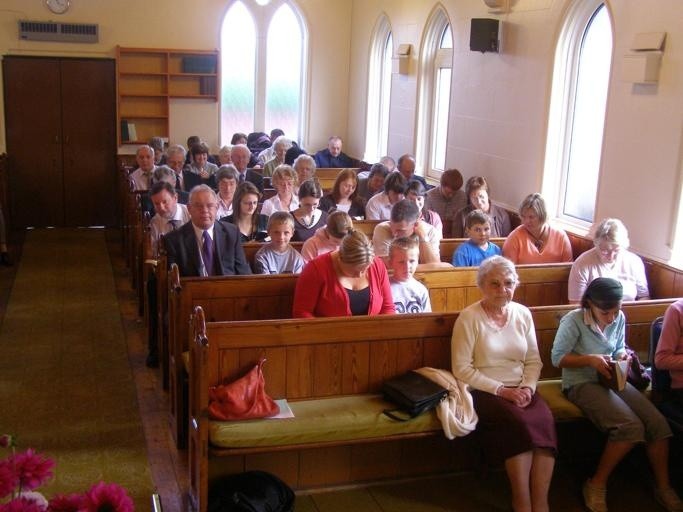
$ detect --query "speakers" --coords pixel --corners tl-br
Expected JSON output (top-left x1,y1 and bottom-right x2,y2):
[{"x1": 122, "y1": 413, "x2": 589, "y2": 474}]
[{"x1": 469, "y1": 17, "x2": 499, "y2": 52}]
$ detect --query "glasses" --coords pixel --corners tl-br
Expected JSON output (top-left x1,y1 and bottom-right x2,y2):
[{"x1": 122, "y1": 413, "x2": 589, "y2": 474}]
[
  {"x1": 487, "y1": 278, "x2": 515, "y2": 287},
  {"x1": 300, "y1": 203, "x2": 320, "y2": 210},
  {"x1": 599, "y1": 246, "x2": 618, "y2": 255}
]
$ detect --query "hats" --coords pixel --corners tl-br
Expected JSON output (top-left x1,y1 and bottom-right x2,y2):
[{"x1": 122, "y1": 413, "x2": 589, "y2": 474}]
[{"x1": 588, "y1": 278, "x2": 622, "y2": 303}]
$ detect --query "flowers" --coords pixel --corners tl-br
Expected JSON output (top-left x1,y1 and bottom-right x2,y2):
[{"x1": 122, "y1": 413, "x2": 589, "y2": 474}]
[{"x1": 0, "y1": 431, "x2": 134, "y2": 511}]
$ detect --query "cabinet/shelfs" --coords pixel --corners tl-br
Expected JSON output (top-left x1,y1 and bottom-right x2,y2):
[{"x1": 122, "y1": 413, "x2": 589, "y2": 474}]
[{"x1": 114, "y1": 43, "x2": 218, "y2": 148}]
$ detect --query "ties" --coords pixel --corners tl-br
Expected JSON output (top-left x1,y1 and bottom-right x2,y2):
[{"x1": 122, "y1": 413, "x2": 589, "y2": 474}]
[
  {"x1": 201, "y1": 230, "x2": 217, "y2": 276},
  {"x1": 239, "y1": 173, "x2": 244, "y2": 184},
  {"x1": 168, "y1": 220, "x2": 178, "y2": 232},
  {"x1": 175, "y1": 174, "x2": 181, "y2": 189}
]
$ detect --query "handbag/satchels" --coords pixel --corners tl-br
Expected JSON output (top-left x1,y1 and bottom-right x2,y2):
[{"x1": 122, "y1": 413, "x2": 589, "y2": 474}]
[
  {"x1": 207, "y1": 358, "x2": 279, "y2": 421},
  {"x1": 205, "y1": 469, "x2": 296, "y2": 512},
  {"x1": 625, "y1": 344, "x2": 652, "y2": 390},
  {"x1": 381, "y1": 370, "x2": 448, "y2": 421}
]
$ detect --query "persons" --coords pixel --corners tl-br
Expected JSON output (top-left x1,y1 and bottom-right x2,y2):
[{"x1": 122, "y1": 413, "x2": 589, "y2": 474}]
[
  {"x1": 549, "y1": 279, "x2": 683, "y2": 511},
  {"x1": 319, "y1": 154, "x2": 574, "y2": 270},
  {"x1": 451, "y1": 253, "x2": 558, "y2": 512},
  {"x1": 654, "y1": 297, "x2": 682, "y2": 425},
  {"x1": 293, "y1": 228, "x2": 396, "y2": 321},
  {"x1": 567, "y1": 216, "x2": 651, "y2": 304},
  {"x1": 382, "y1": 238, "x2": 433, "y2": 315},
  {"x1": 127, "y1": 128, "x2": 353, "y2": 277}
]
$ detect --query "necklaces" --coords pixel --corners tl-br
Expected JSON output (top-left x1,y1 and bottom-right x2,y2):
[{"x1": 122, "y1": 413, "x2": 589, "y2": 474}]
[{"x1": 483, "y1": 301, "x2": 510, "y2": 330}]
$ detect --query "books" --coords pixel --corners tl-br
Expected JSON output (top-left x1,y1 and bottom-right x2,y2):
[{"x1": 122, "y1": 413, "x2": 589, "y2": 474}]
[
  {"x1": 598, "y1": 358, "x2": 629, "y2": 391},
  {"x1": 122, "y1": 121, "x2": 137, "y2": 143},
  {"x1": 181, "y1": 55, "x2": 216, "y2": 95}
]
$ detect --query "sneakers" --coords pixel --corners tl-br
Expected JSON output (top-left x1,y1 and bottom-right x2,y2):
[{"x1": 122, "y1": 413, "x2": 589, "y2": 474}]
[
  {"x1": 653, "y1": 484, "x2": 682, "y2": 512},
  {"x1": 582, "y1": 477, "x2": 607, "y2": 512}
]
